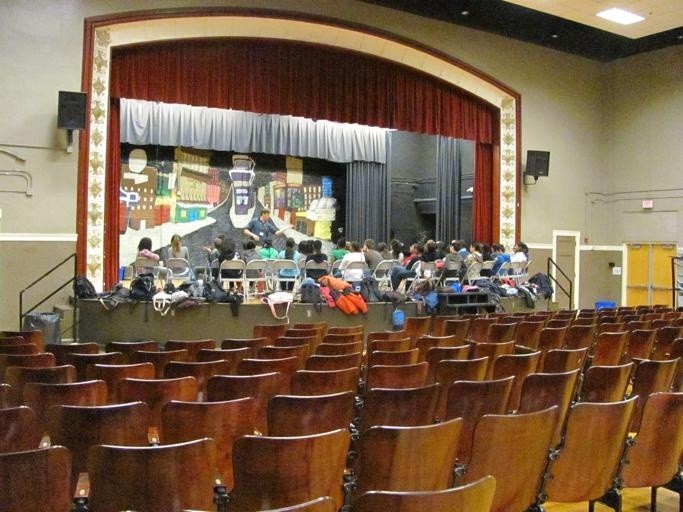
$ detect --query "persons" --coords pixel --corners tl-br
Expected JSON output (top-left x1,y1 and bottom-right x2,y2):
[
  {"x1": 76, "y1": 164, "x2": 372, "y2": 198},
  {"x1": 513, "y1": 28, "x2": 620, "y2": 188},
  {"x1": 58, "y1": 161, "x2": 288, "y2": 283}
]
[
  {"x1": 136, "y1": 235, "x2": 529, "y2": 291},
  {"x1": 243, "y1": 209, "x2": 294, "y2": 253}
]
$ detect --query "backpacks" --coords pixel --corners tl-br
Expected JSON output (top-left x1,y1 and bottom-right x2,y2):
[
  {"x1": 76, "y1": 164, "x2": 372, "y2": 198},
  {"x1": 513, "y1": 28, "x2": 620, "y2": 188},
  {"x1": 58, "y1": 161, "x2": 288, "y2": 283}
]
[{"x1": 300, "y1": 283, "x2": 323, "y2": 313}]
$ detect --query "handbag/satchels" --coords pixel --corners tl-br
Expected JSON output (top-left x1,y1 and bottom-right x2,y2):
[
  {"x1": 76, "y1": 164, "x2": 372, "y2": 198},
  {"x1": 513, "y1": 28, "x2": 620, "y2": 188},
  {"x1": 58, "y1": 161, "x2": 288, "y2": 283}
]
[
  {"x1": 261, "y1": 290, "x2": 294, "y2": 320},
  {"x1": 72, "y1": 273, "x2": 244, "y2": 317}
]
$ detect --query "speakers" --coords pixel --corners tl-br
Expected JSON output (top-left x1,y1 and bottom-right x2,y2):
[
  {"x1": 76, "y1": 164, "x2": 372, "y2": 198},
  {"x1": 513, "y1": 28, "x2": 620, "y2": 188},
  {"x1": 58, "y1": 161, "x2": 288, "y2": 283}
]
[
  {"x1": 57, "y1": 91, "x2": 87, "y2": 130},
  {"x1": 526, "y1": 150, "x2": 550, "y2": 175}
]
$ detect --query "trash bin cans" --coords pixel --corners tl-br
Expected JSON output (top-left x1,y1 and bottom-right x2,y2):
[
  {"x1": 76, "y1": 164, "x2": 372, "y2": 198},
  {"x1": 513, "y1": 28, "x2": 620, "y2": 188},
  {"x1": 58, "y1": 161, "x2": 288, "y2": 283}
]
[{"x1": 24, "y1": 312, "x2": 59, "y2": 349}]
